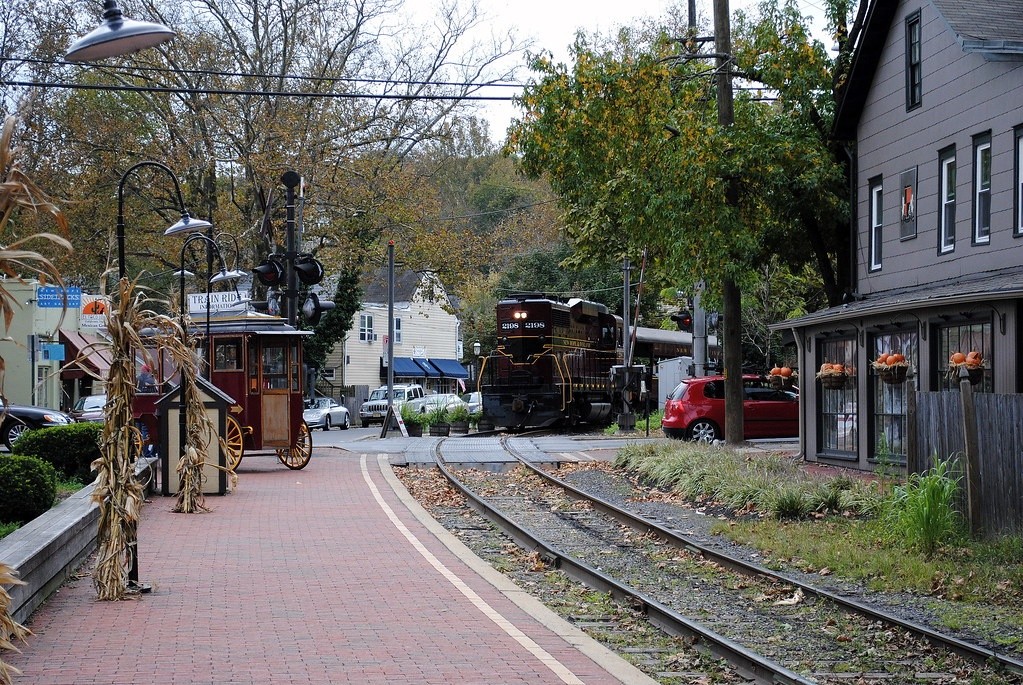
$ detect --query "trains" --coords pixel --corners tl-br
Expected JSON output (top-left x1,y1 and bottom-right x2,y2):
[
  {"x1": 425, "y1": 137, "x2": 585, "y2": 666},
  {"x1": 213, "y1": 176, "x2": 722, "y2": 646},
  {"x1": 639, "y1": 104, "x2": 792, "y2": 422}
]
[{"x1": 472, "y1": 292, "x2": 724, "y2": 434}]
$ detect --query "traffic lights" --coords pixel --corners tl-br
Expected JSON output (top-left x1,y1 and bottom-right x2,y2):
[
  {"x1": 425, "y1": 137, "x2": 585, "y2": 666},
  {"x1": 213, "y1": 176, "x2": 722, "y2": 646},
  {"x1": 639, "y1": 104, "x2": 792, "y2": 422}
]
[
  {"x1": 668, "y1": 310, "x2": 696, "y2": 332},
  {"x1": 707, "y1": 311, "x2": 724, "y2": 329},
  {"x1": 251, "y1": 258, "x2": 285, "y2": 287},
  {"x1": 297, "y1": 258, "x2": 338, "y2": 328}
]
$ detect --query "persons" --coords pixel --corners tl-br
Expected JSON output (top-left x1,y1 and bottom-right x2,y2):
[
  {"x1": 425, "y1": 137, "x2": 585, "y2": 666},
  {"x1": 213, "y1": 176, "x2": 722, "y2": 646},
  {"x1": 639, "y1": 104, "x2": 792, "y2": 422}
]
[{"x1": 138, "y1": 364, "x2": 158, "y2": 392}]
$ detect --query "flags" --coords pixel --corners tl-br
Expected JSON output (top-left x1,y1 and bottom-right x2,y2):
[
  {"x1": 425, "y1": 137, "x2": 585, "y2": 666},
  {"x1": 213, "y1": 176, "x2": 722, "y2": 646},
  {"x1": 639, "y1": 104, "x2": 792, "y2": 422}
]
[{"x1": 459, "y1": 379, "x2": 466, "y2": 392}]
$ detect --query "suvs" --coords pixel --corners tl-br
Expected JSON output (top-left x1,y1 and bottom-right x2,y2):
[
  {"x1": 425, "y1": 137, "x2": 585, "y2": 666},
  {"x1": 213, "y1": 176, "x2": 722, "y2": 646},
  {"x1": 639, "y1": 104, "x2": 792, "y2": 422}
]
[{"x1": 360, "y1": 382, "x2": 428, "y2": 424}]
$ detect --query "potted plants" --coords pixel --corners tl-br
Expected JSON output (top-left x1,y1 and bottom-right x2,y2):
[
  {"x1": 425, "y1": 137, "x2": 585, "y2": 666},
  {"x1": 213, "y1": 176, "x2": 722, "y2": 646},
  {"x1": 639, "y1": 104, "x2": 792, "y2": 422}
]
[
  {"x1": 449, "y1": 401, "x2": 473, "y2": 435},
  {"x1": 472, "y1": 408, "x2": 495, "y2": 432},
  {"x1": 400, "y1": 402, "x2": 429, "y2": 437},
  {"x1": 426, "y1": 395, "x2": 454, "y2": 436}
]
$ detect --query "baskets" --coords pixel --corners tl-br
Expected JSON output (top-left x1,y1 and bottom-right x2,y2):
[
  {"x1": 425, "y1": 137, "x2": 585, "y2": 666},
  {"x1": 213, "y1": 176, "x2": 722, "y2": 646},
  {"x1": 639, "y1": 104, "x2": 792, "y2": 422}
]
[
  {"x1": 876, "y1": 365, "x2": 908, "y2": 384},
  {"x1": 770, "y1": 376, "x2": 795, "y2": 390},
  {"x1": 822, "y1": 374, "x2": 847, "y2": 390},
  {"x1": 950, "y1": 366, "x2": 985, "y2": 385}
]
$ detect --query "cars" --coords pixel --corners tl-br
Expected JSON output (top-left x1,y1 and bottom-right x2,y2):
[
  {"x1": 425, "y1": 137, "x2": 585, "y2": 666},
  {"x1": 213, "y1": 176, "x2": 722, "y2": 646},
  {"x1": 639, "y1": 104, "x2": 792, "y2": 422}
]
[
  {"x1": 658, "y1": 374, "x2": 800, "y2": 443},
  {"x1": 0, "y1": 395, "x2": 77, "y2": 455},
  {"x1": 67, "y1": 393, "x2": 109, "y2": 425},
  {"x1": 836, "y1": 402, "x2": 858, "y2": 443},
  {"x1": 459, "y1": 391, "x2": 483, "y2": 416},
  {"x1": 111, "y1": 299, "x2": 313, "y2": 482},
  {"x1": 418, "y1": 394, "x2": 470, "y2": 418},
  {"x1": 300, "y1": 397, "x2": 351, "y2": 430}
]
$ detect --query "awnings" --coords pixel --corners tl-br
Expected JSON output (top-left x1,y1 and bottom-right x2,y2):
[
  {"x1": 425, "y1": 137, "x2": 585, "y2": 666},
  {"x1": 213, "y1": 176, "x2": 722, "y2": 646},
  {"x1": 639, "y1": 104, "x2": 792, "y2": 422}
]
[
  {"x1": 394, "y1": 357, "x2": 425, "y2": 376},
  {"x1": 428, "y1": 358, "x2": 468, "y2": 378},
  {"x1": 413, "y1": 357, "x2": 440, "y2": 377},
  {"x1": 59, "y1": 330, "x2": 116, "y2": 380}
]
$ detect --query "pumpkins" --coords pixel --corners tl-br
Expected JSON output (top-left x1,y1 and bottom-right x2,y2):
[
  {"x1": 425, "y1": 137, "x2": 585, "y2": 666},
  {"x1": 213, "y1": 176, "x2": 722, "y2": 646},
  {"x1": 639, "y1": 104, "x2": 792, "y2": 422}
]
[
  {"x1": 951, "y1": 352, "x2": 982, "y2": 365},
  {"x1": 771, "y1": 363, "x2": 792, "y2": 376},
  {"x1": 821, "y1": 363, "x2": 844, "y2": 372},
  {"x1": 877, "y1": 353, "x2": 905, "y2": 365}
]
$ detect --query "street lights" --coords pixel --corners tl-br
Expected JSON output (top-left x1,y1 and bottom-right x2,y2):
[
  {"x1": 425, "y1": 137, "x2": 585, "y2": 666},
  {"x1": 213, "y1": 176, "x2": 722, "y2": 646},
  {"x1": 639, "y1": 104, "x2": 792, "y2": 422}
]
[
  {"x1": 173, "y1": 230, "x2": 250, "y2": 495},
  {"x1": 114, "y1": 160, "x2": 215, "y2": 335},
  {"x1": 473, "y1": 338, "x2": 482, "y2": 390}
]
